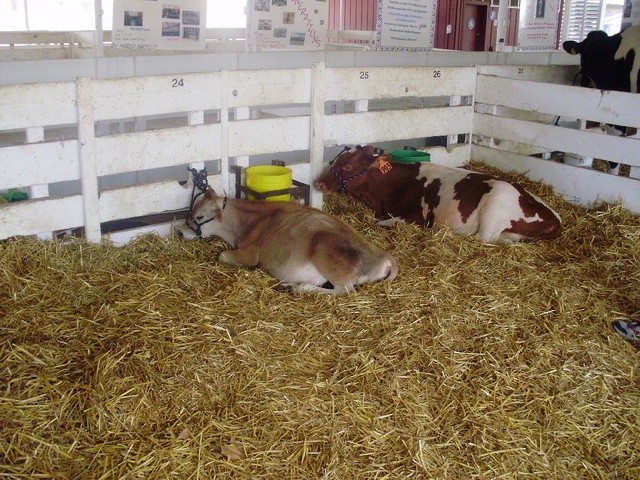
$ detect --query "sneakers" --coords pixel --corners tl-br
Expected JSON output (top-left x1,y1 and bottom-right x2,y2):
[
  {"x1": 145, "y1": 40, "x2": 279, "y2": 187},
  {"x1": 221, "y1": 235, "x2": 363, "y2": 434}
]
[{"x1": 613, "y1": 318, "x2": 640, "y2": 341}]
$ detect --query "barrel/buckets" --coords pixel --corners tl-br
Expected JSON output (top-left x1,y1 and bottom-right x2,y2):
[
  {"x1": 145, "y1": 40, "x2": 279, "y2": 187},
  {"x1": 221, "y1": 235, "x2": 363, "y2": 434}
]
[
  {"x1": 390, "y1": 151, "x2": 431, "y2": 163},
  {"x1": 246, "y1": 165, "x2": 292, "y2": 201}
]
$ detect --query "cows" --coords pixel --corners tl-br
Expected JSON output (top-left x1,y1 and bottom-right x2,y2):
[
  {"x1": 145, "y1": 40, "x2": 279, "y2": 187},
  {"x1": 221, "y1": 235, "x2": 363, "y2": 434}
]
[
  {"x1": 177, "y1": 183, "x2": 400, "y2": 297},
  {"x1": 313, "y1": 142, "x2": 563, "y2": 244},
  {"x1": 562, "y1": 24, "x2": 640, "y2": 176}
]
